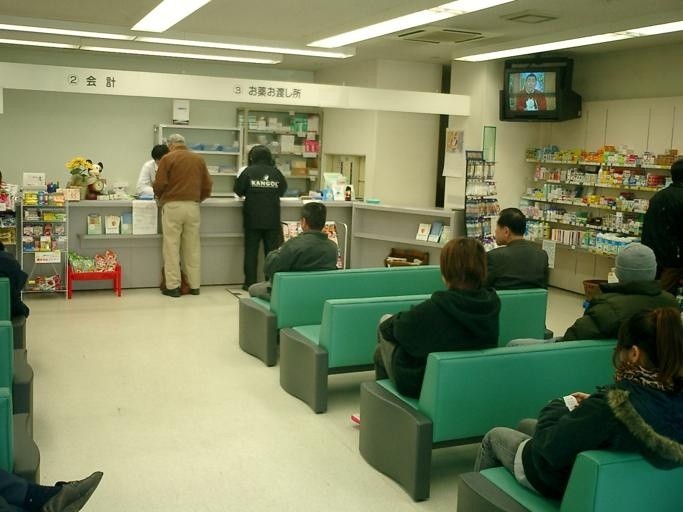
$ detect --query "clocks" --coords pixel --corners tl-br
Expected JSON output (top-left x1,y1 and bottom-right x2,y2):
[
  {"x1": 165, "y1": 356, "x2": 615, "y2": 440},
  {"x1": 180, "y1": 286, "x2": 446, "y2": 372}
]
[{"x1": 85, "y1": 159, "x2": 107, "y2": 200}]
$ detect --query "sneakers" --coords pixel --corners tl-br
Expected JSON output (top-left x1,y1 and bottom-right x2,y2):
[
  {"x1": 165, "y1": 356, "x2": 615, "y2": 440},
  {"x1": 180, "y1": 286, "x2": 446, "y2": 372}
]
[
  {"x1": 190, "y1": 288, "x2": 200, "y2": 295},
  {"x1": 161, "y1": 288, "x2": 182, "y2": 297}
]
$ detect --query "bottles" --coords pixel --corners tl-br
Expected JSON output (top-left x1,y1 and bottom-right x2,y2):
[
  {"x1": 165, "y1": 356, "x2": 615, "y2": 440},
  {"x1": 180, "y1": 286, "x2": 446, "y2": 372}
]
[
  {"x1": 544, "y1": 223, "x2": 551, "y2": 239},
  {"x1": 38, "y1": 191, "x2": 43, "y2": 204},
  {"x1": 345, "y1": 186, "x2": 352, "y2": 200},
  {"x1": 44, "y1": 193, "x2": 49, "y2": 204},
  {"x1": 539, "y1": 223, "x2": 544, "y2": 240}
]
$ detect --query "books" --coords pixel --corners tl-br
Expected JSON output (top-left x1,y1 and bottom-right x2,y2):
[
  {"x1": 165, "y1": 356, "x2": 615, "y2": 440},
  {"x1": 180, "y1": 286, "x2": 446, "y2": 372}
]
[{"x1": 416, "y1": 221, "x2": 450, "y2": 243}]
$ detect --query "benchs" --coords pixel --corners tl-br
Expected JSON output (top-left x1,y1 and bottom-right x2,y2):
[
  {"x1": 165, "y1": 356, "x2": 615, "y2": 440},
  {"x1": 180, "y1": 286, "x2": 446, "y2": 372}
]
[
  {"x1": 455, "y1": 442, "x2": 683, "y2": 512},
  {"x1": 0, "y1": 321, "x2": 33, "y2": 415},
  {"x1": 279, "y1": 287, "x2": 554, "y2": 414},
  {"x1": 0, "y1": 277, "x2": 26, "y2": 350},
  {"x1": 358, "y1": 338, "x2": 623, "y2": 503},
  {"x1": 238, "y1": 265, "x2": 451, "y2": 367},
  {"x1": 0, "y1": 386, "x2": 41, "y2": 487}
]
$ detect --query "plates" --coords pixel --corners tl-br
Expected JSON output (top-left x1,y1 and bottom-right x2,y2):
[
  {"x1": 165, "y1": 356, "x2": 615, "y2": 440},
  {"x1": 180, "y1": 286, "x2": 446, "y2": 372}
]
[{"x1": 365, "y1": 199, "x2": 380, "y2": 203}]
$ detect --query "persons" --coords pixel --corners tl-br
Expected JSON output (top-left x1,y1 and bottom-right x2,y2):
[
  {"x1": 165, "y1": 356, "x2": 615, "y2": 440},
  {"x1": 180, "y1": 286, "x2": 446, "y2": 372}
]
[
  {"x1": 641, "y1": 159, "x2": 683, "y2": 295},
  {"x1": 153, "y1": 134, "x2": 213, "y2": 297},
  {"x1": 351, "y1": 235, "x2": 502, "y2": 424},
  {"x1": 473, "y1": 307, "x2": 683, "y2": 500},
  {"x1": 484, "y1": 208, "x2": 550, "y2": 292},
  {"x1": 233, "y1": 145, "x2": 288, "y2": 291},
  {"x1": 136, "y1": 144, "x2": 170, "y2": 197},
  {"x1": 516, "y1": 73, "x2": 547, "y2": 112},
  {"x1": 248, "y1": 202, "x2": 339, "y2": 302},
  {"x1": 0, "y1": 470, "x2": 103, "y2": 512},
  {"x1": 0, "y1": 239, "x2": 29, "y2": 320},
  {"x1": 507, "y1": 242, "x2": 683, "y2": 347}
]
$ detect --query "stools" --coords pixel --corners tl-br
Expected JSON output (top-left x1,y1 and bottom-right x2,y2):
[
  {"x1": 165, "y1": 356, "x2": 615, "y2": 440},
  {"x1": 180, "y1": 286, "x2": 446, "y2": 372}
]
[{"x1": 65, "y1": 262, "x2": 122, "y2": 300}]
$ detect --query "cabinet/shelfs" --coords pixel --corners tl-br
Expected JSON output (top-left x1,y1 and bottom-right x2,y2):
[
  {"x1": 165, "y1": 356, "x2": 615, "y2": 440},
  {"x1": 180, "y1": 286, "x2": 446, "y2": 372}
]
[
  {"x1": 236, "y1": 108, "x2": 324, "y2": 197},
  {"x1": 520, "y1": 158, "x2": 673, "y2": 295},
  {"x1": 68, "y1": 200, "x2": 353, "y2": 292},
  {"x1": 151, "y1": 124, "x2": 243, "y2": 199},
  {"x1": 20, "y1": 196, "x2": 69, "y2": 302},
  {"x1": 0, "y1": 183, "x2": 20, "y2": 263},
  {"x1": 463, "y1": 158, "x2": 500, "y2": 251},
  {"x1": 350, "y1": 201, "x2": 465, "y2": 268}
]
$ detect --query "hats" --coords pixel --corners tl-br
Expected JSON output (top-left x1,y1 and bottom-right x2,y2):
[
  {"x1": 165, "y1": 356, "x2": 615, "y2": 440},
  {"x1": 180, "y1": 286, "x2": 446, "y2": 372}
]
[{"x1": 614, "y1": 242, "x2": 658, "y2": 285}]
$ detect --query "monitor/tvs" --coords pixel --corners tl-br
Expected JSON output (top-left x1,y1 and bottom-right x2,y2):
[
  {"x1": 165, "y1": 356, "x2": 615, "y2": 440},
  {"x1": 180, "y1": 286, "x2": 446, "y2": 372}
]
[{"x1": 503, "y1": 65, "x2": 583, "y2": 122}]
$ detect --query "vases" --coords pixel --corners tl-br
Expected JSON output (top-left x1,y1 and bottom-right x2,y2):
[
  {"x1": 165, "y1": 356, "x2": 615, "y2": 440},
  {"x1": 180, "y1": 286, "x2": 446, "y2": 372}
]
[{"x1": 69, "y1": 185, "x2": 88, "y2": 200}]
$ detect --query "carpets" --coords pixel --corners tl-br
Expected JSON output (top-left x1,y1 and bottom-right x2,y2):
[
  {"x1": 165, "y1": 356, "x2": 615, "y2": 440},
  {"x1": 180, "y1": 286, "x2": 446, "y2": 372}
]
[{"x1": 226, "y1": 287, "x2": 251, "y2": 299}]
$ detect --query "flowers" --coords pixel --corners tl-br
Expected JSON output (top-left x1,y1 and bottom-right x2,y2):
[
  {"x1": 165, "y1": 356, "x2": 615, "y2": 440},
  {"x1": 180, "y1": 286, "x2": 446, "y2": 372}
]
[{"x1": 63, "y1": 157, "x2": 94, "y2": 184}]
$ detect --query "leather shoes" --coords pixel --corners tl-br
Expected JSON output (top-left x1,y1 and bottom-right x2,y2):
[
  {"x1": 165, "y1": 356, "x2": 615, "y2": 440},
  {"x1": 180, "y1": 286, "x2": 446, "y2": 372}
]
[{"x1": 42, "y1": 471, "x2": 104, "y2": 512}]
